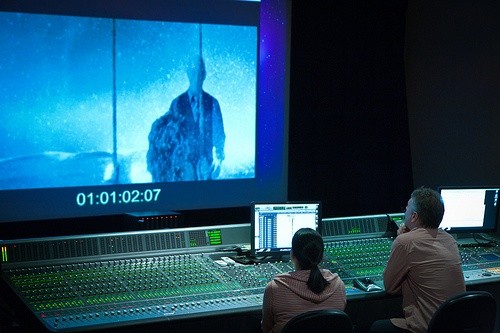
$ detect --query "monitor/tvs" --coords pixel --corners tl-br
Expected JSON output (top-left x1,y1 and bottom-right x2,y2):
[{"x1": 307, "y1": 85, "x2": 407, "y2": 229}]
[
  {"x1": 437, "y1": 186, "x2": 500, "y2": 233},
  {"x1": 251, "y1": 201, "x2": 322, "y2": 262}
]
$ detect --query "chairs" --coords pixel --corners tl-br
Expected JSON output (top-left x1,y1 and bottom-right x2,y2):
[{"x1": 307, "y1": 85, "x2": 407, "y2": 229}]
[
  {"x1": 426, "y1": 289, "x2": 497, "y2": 333},
  {"x1": 281, "y1": 308, "x2": 353, "y2": 333}
]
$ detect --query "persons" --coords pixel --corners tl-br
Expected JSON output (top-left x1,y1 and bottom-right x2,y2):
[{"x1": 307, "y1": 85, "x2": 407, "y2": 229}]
[
  {"x1": 168, "y1": 54, "x2": 225, "y2": 181},
  {"x1": 371, "y1": 187, "x2": 467, "y2": 333},
  {"x1": 262, "y1": 227, "x2": 347, "y2": 333}
]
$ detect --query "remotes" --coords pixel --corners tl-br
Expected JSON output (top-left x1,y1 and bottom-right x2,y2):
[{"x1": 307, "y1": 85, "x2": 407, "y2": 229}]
[
  {"x1": 221, "y1": 257, "x2": 235, "y2": 265},
  {"x1": 215, "y1": 260, "x2": 227, "y2": 267}
]
